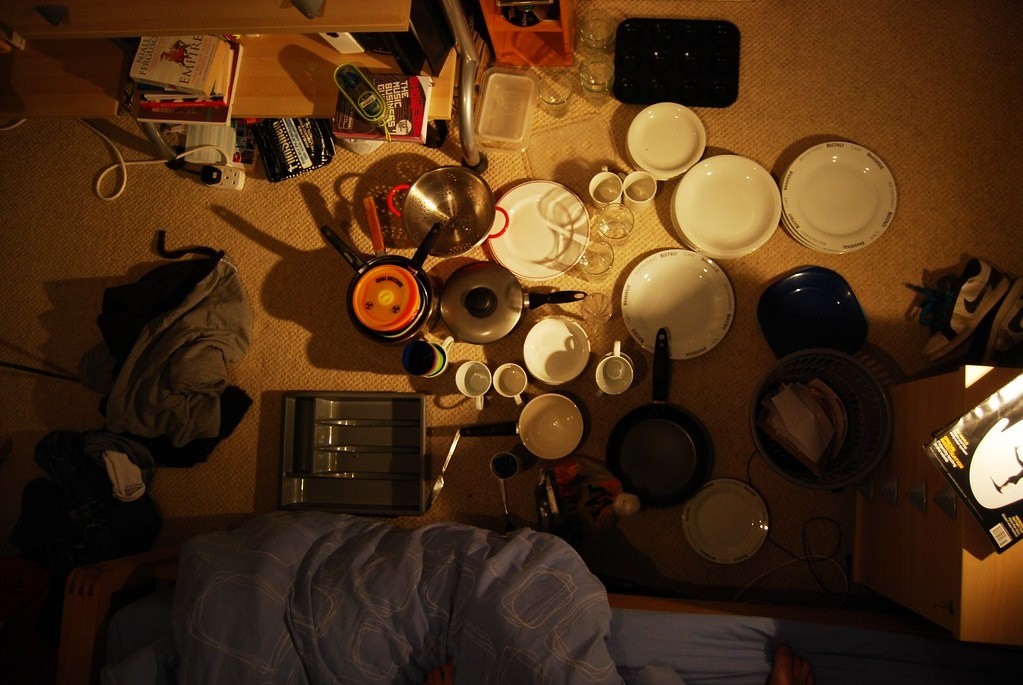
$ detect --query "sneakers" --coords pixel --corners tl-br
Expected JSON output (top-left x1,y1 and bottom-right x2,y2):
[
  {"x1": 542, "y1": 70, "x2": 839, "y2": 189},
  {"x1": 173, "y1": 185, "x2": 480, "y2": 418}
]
[
  {"x1": 983, "y1": 271, "x2": 1023, "y2": 364},
  {"x1": 906, "y1": 249, "x2": 1010, "y2": 361}
]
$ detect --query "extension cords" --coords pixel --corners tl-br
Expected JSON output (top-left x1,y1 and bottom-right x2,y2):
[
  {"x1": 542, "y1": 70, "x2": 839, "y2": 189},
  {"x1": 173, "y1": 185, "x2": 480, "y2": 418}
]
[{"x1": 206, "y1": 164, "x2": 246, "y2": 191}]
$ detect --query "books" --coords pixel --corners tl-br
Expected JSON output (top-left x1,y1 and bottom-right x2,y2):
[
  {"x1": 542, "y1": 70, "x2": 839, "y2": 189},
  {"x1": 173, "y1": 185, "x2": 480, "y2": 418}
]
[
  {"x1": 923, "y1": 372, "x2": 1023, "y2": 555},
  {"x1": 130, "y1": 36, "x2": 243, "y2": 126},
  {"x1": 332, "y1": 74, "x2": 433, "y2": 144}
]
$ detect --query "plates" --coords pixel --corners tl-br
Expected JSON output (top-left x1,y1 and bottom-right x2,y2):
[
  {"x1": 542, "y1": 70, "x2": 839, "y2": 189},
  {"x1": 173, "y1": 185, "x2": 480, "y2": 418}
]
[
  {"x1": 668, "y1": 154, "x2": 782, "y2": 259},
  {"x1": 625, "y1": 101, "x2": 706, "y2": 181},
  {"x1": 757, "y1": 264, "x2": 868, "y2": 363},
  {"x1": 488, "y1": 178, "x2": 590, "y2": 282},
  {"x1": 614, "y1": 17, "x2": 741, "y2": 108},
  {"x1": 779, "y1": 141, "x2": 897, "y2": 256},
  {"x1": 621, "y1": 249, "x2": 735, "y2": 360},
  {"x1": 682, "y1": 479, "x2": 769, "y2": 564}
]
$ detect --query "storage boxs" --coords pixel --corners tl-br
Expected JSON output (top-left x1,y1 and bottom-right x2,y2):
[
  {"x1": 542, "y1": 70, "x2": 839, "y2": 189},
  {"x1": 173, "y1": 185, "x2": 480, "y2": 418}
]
[{"x1": 472, "y1": 66, "x2": 540, "y2": 155}]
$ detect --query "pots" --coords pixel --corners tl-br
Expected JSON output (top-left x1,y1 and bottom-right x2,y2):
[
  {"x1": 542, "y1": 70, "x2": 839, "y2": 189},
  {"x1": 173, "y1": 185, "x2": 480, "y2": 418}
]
[
  {"x1": 460, "y1": 390, "x2": 591, "y2": 451},
  {"x1": 605, "y1": 327, "x2": 715, "y2": 510},
  {"x1": 320, "y1": 196, "x2": 444, "y2": 345},
  {"x1": 439, "y1": 262, "x2": 588, "y2": 344}
]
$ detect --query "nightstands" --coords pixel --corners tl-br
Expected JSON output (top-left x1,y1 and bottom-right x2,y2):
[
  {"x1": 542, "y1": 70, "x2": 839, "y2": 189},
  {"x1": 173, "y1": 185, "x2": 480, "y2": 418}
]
[{"x1": 852, "y1": 366, "x2": 1023, "y2": 647}]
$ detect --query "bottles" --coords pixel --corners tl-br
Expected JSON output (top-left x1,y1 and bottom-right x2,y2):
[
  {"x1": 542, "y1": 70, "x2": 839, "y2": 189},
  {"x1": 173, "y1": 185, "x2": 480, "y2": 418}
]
[
  {"x1": 340, "y1": 68, "x2": 385, "y2": 123},
  {"x1": 551, "y1": 457, "x2": 641, "y2": 529}
]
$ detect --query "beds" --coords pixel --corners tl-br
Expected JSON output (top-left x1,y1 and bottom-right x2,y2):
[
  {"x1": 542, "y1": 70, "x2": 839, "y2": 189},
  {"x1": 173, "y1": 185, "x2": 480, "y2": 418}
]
[{"x1": 56, "y1": 555, "x2": 1023, "y2": 685}]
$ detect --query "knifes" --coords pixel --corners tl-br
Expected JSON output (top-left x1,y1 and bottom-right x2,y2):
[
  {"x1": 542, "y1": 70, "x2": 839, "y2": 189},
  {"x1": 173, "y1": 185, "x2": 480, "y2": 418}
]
[
  {"x1": 425, "y1": 429, "x2": 461, "y2": 505},
  {"x1": 545, "y1": 475, "x2": 562, "y2": 543}
]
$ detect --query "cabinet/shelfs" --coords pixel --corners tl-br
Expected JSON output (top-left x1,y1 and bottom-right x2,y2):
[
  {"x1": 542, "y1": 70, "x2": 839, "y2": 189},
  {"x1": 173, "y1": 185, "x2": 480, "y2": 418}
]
[
  {"x1": 478, "y1": 0, "x2": 577, "y2": 66},
  {"x1": 0, "y1": 0, "x2": 456, "y2": 164}
]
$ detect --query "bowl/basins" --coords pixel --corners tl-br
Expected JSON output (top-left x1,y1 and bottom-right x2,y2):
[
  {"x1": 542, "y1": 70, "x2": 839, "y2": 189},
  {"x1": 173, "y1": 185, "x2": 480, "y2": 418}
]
[
  {"x1": 518, "y1": 393, "x2": 583, "y2": 459},
  {"x1": 524, "y1": 317, "x2": 591, "y2": 386},
  {"x1": 750, "y1": 349, "x2": 894, "y2": 489},
  {"x1": 352, "y1": 264, "x2": 422, "y2": 332},
  {"x1": 473, "y1": 65, "x2": 538, "y2": 153}
]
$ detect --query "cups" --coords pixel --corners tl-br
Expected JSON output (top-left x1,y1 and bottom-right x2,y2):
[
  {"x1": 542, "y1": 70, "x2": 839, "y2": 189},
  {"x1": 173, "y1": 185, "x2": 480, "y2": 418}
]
[
  {"x1": 455, "y1": 361, "x2": 492, "y2": 411},
  {"x1": 580, "y1": 52, "x2": 615, "y2": 106},
  {"x1": 595, "y1": 340, "x2": 633, "y2": 395},
  {"x1": 581, "y1": 293, "x2": 612, "y2": 325},
  {"x1": 493, "y1": 363, "x2": 528, "y2": 405},
  {"x1": 580, "y1": 9, "x2": 613, "y2": 51},
  {"x1": 588, "y1": 162, "x2": 623, "y2": 206},
  {"x1": 624, "y1": 170, "x2": 656, "y2": 213},
  {"x1": 538, "y1": 73, "x2": 571, "y2": 118},
  {"x1": 400, "y1": 337, "x2": 444, "y2": 377},
  {"x1": 580, "y1": 240, "x2": 616, "y2": 284},
  {"x1": 489, "y1": 452, "x2": 518, "y2": 479},
  {"x1": 596, "y1": 203, "x2": 633, "y2": 240},
  {"x1": 424, "y1": 335, "x2": 454, "y2": 378},
  {"x1": 596, "y1": 351, "x2": 633, "y2": 398}
]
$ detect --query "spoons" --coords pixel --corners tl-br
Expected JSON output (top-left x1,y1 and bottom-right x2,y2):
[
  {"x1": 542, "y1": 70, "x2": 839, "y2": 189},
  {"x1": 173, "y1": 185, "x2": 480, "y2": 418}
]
[{"x1": 497, "y1": 479, "x2": 516, "y2": 534}]
